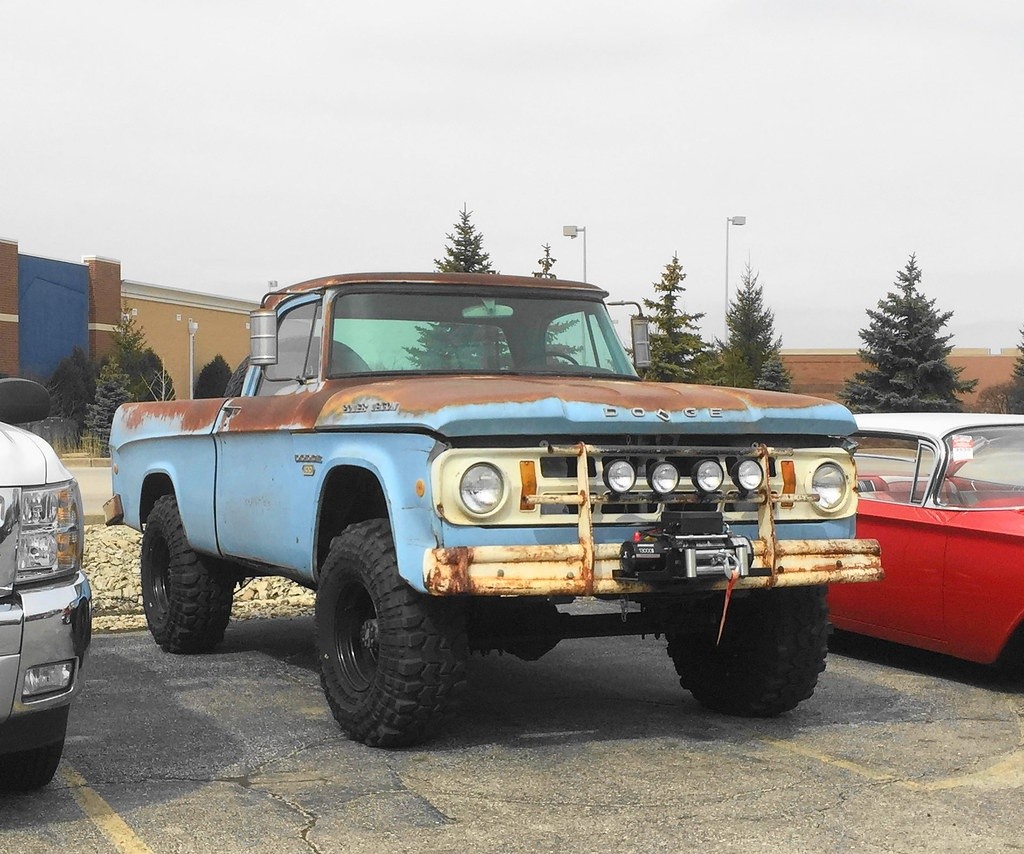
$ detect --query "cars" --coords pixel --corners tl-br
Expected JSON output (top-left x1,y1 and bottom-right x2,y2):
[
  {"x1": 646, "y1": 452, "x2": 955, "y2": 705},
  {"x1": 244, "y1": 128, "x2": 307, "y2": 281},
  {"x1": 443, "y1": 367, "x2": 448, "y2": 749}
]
[{"x1": 829, "y1": 410, "x2": 1024, "y2": 673}]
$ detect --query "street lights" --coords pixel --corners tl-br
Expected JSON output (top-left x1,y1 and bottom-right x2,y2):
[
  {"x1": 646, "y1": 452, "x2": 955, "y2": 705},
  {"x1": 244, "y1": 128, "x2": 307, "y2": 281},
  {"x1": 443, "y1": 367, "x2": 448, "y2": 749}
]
[
  {"x1": 724, "y1": 214, "x2": 746, "y2": 351},
  {"x1": 561, "y1": 226, "x2": 589, "y2": 286}
]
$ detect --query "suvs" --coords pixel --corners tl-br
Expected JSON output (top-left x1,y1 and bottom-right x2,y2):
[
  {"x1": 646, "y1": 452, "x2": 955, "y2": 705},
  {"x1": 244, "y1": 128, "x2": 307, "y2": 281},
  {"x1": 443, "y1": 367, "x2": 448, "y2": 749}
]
[{"x1": 0, "y1": 378, "x2": 91, "y2": 790}]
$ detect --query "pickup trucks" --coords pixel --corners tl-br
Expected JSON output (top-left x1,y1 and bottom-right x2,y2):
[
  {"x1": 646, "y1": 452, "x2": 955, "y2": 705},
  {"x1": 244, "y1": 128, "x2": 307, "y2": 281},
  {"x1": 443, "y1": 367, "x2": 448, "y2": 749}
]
[{"x1": 105, "y1": 271, "x2": 881, "y2": 744}]
